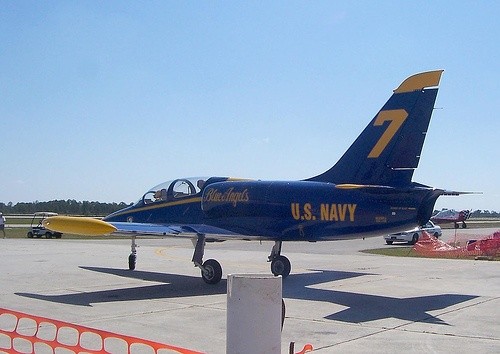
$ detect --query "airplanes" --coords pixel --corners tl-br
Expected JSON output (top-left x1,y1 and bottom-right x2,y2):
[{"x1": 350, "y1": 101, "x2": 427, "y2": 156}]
[
  {"x1": 429, "y1": 209, "x2": 474, "y2": 229},
  {"x1": 41, "y1": 69, "x2": 484, "y2": 283}
]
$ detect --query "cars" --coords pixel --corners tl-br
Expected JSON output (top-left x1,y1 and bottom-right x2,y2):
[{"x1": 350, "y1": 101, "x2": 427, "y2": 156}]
[
  {"x1": 26, "y1": 212, "x2": 64, "y2": 238},
  {"x1": 383, "y1": 219, "x2": 443, "y2": 245}
]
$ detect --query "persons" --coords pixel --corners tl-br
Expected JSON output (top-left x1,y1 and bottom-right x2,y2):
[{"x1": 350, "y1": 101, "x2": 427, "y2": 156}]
[{"x1": 0, "y1": 212, "x2": 6, "y2": 239}]
